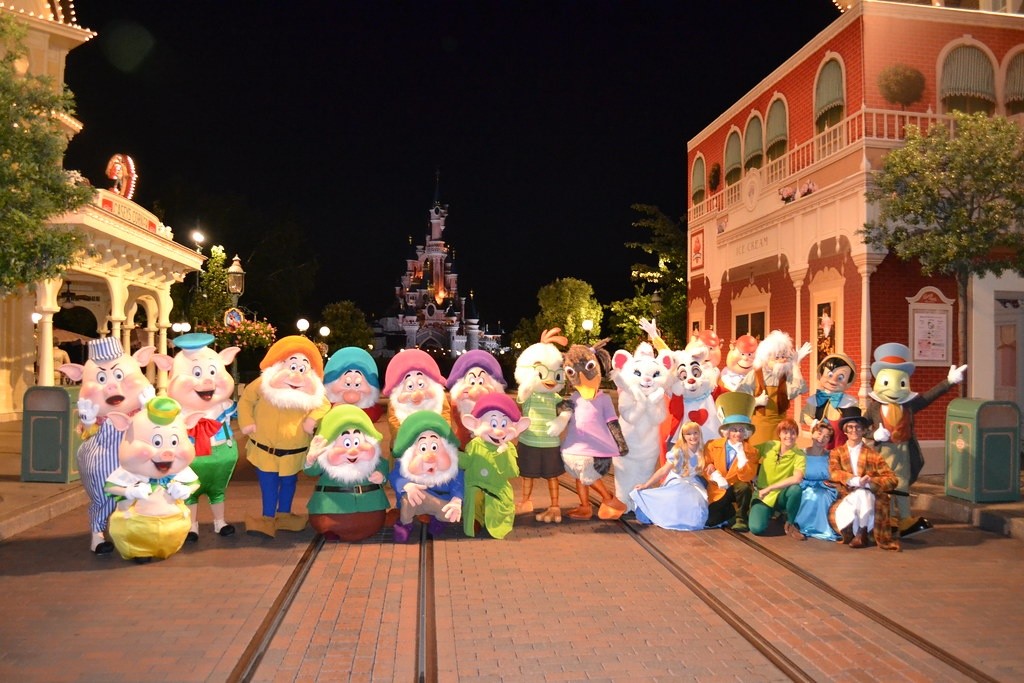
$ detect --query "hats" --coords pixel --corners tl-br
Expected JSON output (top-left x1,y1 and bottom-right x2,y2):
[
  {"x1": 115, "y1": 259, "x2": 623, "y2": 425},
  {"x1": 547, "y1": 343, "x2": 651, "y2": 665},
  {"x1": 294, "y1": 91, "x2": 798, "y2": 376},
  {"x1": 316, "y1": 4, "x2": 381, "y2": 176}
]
[
  {"x1": 838, "y1": 406, "x2": 869, "y2": 432},
  {"x1": 716, "y1": 392, "x2": 756, "y2": 439}
]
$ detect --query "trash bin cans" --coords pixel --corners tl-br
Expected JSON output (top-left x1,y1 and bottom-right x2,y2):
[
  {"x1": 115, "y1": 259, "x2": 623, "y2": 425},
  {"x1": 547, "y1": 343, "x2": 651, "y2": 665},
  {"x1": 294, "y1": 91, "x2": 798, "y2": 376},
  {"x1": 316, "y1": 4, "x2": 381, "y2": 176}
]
[
  {"x1": 944, "y1": 397, "x2": 1019, "y2": 502},
  {"x1": 21, "y1": 385, "x2": 82, "y2": 483}
]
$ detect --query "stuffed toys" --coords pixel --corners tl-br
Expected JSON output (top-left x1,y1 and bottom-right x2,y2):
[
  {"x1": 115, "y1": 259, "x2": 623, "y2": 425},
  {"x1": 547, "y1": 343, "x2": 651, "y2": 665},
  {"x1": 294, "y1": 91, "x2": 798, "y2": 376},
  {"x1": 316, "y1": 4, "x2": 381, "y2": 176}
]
[
  {"x1": 864, "y1": 343, "x2": 967, "y2": 538},
  {"x1": 515, "y1": 327, "x2": 721, "y2": 522},
  {"x1": 59, "y1": 332, "x2": 241, "y2": 563}
]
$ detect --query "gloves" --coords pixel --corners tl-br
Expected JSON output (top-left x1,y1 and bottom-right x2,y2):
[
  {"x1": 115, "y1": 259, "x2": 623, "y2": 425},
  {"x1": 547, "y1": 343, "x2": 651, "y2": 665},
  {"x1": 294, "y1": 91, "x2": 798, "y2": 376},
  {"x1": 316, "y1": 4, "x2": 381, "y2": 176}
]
[
  {"x1": 736, "y1": 443, "x2": 749, "y2": 468},
  {"x1": 710, "y1": 469, "x2": 728, "y2": 490}
]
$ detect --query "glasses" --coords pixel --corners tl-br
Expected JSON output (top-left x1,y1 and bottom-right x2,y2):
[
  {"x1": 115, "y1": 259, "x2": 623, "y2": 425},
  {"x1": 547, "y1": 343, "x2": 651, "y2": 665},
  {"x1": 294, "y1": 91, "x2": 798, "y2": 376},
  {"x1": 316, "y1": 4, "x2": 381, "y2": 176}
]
[
  {"x1": 846, "y1": 425, "x2": 864, "y2": 431},
  {"x1": 729, "y1": 428, "x2": 745, "y2": 433}
]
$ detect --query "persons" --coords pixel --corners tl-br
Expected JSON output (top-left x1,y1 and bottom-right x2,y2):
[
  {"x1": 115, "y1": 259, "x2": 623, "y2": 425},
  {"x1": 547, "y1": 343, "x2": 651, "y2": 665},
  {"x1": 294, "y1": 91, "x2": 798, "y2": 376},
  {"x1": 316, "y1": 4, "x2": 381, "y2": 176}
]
[
  {"x1": 639, "y1": 317, "x2": 812, "y2": 446},
  {"x1": 827, "y1": 407, "x2": 900, "y2": 550},
  {"x1": 631, "y1": 422, "x2": 716, "y2": 531},
  {"x1": 706, "y1": 392, "x2": 758, "y2": 532},
  {"x1": 801, "y1": 353, "x2": 858, "y2": 449},
  {"x1": 798, "y1": 423, "x2": 843, "y2": 542},
  {"x1": 750, "y1": 418, "x2": 806, "y2": 540},
  {"x1": 237, "y1": 336, "x2": 531, "y2": 539},
  {"x1": 53, "y1": 336, "x2": 71, "y2": 385}
]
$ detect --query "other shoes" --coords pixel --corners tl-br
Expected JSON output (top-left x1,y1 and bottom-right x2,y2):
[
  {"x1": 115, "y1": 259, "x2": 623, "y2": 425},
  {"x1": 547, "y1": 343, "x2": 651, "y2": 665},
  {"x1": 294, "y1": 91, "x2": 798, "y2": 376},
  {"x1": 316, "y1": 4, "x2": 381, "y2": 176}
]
[
  {"x1": 784, "y1": 522, "x2": 806, "y2": 540},
  {"x1": 731, "y1": 514, "x2": 749, "y2": 532},
  {"x1": 849, "y1": 526, "x2": 870, "y2": 548}
]
